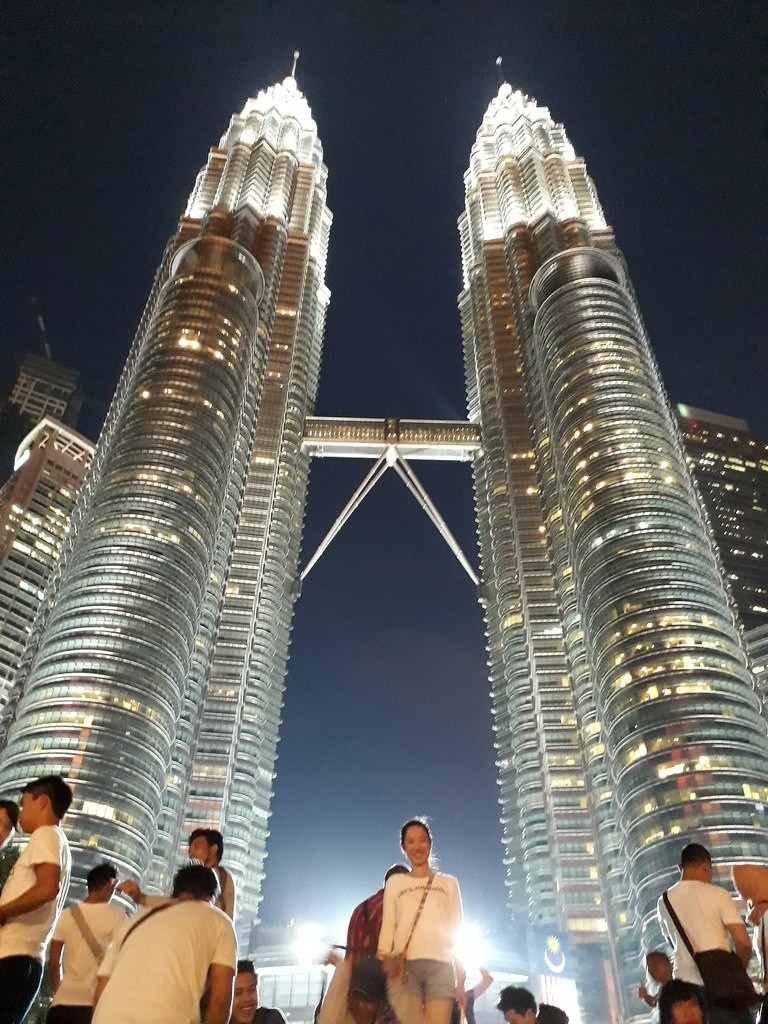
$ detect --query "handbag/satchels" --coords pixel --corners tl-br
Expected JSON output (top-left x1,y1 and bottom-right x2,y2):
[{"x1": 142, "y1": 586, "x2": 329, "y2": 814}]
[
  {"x1": 693, "y1": 949, "x2": 760, "y2": 1007},
  {"x1": 379, "y1": 951, "x2": 407, "y2": 978},
  {"x1": 460, "y1": 1007, "x2": 468, "y2": 1024}
]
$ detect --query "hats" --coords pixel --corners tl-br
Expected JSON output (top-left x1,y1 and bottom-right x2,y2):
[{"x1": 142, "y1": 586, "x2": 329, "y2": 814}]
[{"x1": 350, "y1": 957, "x2": 386, "y2": 1001}]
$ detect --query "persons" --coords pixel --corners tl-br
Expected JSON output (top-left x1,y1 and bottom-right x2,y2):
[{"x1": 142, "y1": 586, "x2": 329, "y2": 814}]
[
  {"x1": 117, "y1": 829, "x2": 233, "y2": 922},
  {"x1": 229, "y1": 960, "x2": 288, "y2": 1024},
  {"x1": 0, "y1": 775, "x2": 73, "y2": 1024},
  {"x1": 638, "y1": 844, "x2": 768, "y2": 1024},
  {"x1": 90, "y1": 862, "x2": 238, "y2": 1024},
  {"x1": 45, "y1": 864, "x2": 125, "y2": 1024},
  {"x1": 496, "y1": 987, "x2": 568, "y2": 1024},
  {"x1": 315, "y1": 819, "x2": 493, "y2": 1024}
]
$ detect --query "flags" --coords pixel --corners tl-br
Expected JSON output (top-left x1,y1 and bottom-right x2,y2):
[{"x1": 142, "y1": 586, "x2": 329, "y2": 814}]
[{"x1": 535, "y1": 929, "x2": 581, "y2": 1024}]
